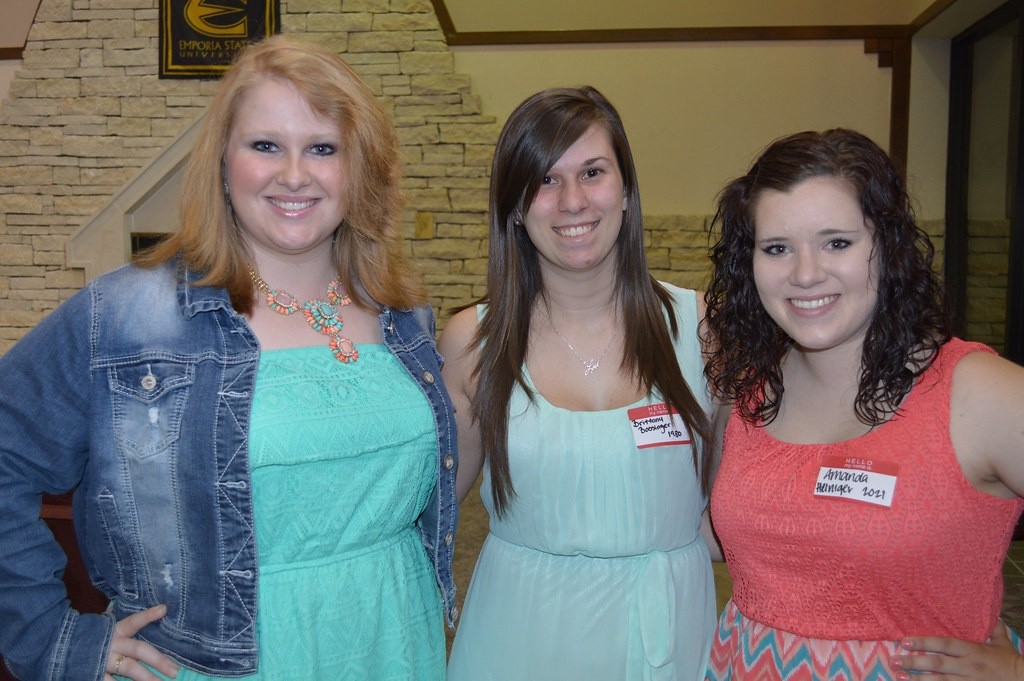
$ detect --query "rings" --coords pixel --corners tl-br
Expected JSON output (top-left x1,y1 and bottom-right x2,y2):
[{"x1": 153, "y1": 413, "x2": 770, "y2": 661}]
[{"x1": 115, "y1": 656, "x2": 124, "y2": 674}]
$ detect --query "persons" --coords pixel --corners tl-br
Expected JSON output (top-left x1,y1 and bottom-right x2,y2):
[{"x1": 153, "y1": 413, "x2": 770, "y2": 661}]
[
  {"x1": 438, "y1": 85, "x2": 744, "y2": 681},
  {"x1": 697, "y1": 128, "x2": 1024, "y2": 681},
  {"x1": 0, "y1": 38, "x2": 460, "y2": 681}
]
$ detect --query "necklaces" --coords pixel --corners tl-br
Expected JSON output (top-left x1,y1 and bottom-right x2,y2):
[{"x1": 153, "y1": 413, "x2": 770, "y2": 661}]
[
  {"x1": 248, "y1": 269, "x2": 358, "y2": 363},
  {"x1": 541, "y1": 308, "x2": 618, "y2": 376}
]
